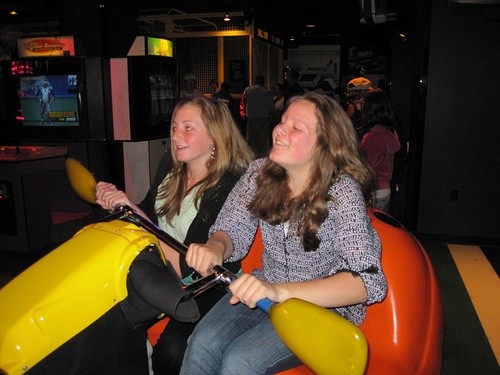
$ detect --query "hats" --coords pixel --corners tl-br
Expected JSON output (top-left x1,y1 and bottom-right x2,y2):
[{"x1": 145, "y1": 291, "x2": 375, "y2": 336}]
[
  {"x1": 346, "y1": 77, "x2": 373, "y2": 91},
  {"x1": 183, "y1": 74, "x2": 196, "y2": 80}
]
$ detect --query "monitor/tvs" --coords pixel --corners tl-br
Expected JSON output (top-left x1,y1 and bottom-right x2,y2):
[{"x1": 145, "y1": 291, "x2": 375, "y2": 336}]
[{"x1": 18, "y1": 74, "x2": 80, "y2": 127}]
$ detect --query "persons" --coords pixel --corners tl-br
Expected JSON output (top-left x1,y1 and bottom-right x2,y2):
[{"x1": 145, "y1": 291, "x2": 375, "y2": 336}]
[
  {"x1": 179, "y1": 91, "x2": 388, "y2": 375},
  {"x1": 34, "y1": 79, "x2": 55, "y2": 126},
  {"x1": 180, "y1": 67, "x2": 401, "y2": 210},
  {"x1": 91, "y1": 95, "x2": 258, "y2": 375}
]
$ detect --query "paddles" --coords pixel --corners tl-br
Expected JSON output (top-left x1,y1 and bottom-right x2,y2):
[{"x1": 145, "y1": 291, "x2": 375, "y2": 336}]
[{"x1": 65, "y1": 157, "x2": 369, "y2": 375}]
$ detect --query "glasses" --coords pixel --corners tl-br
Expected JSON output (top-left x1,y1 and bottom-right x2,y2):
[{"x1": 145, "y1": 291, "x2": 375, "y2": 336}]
[{"x1": 186, "y1": 80, "x2": 196, "y2": 84}]
